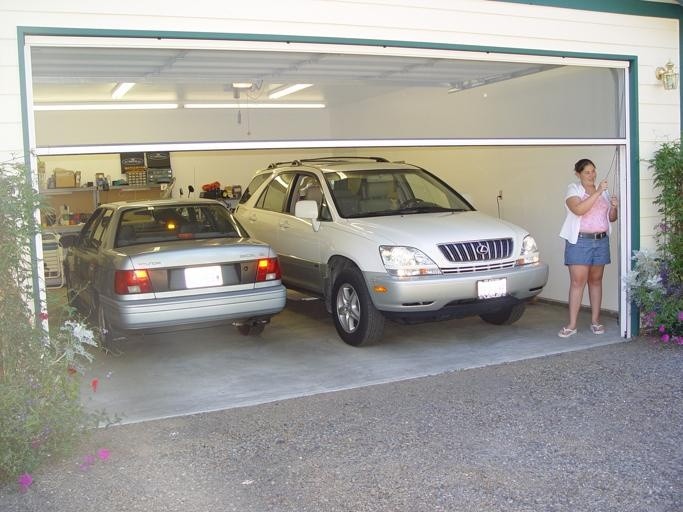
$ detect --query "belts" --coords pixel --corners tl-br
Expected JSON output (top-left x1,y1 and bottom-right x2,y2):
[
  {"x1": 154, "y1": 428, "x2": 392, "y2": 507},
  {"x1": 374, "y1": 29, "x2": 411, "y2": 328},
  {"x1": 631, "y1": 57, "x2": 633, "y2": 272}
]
[{"x1": 578, "y1": 232, "x2": 606, "y2": 240}]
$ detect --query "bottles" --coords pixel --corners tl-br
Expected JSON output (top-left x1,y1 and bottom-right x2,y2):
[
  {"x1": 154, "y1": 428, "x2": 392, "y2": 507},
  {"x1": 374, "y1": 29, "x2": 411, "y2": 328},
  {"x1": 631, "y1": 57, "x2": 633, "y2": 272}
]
[
  {"x1": 63, "y1": 206, "x2": 69, "y2": 226},
  {"x1": 103, "y1": 178, "x2": 109, "y2": 191}
]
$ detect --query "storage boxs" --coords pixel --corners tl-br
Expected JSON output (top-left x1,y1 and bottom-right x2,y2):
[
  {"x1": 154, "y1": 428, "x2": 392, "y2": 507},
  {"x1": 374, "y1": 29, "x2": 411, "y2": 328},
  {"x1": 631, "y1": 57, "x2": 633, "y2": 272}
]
[{"x1": 55, "y1": 168, "x2": 76, "y2": 188}]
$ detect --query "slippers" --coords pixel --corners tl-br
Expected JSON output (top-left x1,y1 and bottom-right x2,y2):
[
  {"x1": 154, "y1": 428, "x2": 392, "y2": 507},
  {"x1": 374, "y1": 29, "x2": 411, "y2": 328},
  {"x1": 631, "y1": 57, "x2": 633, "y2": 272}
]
[
  {"x1": 591, "y1": 323, "x2": 604, "y2": 335},
  {"x1": 558, "y1": 326, "x2": 577, "y2": 338}
]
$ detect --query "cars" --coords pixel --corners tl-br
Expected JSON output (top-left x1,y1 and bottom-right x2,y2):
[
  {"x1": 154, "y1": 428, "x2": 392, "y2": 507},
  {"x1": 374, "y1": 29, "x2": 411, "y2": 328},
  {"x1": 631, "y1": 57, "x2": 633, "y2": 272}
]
[
  {"x1": 234, "y1": 156, "x2": 549, "y2": 347},
  {"x1": 58, "y1": 198, "x2": 287, "y2": 355}
]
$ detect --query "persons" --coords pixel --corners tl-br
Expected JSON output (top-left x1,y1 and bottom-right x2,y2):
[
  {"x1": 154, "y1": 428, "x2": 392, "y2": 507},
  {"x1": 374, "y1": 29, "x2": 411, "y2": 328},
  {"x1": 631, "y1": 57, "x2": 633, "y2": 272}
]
[{"x1": 556, "y1": 158, "x2": 618, "y2": 339}]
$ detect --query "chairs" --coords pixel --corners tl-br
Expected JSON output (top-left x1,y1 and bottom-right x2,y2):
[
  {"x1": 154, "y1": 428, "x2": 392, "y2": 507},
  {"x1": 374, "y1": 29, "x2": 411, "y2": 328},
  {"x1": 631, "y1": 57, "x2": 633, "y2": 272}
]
[
  {"x1": 302, "y1": 176, "x2": 402, "y2": 221},
  {"x1": 118, "y1": 219, "x2": 214, "y2": 246}
]
